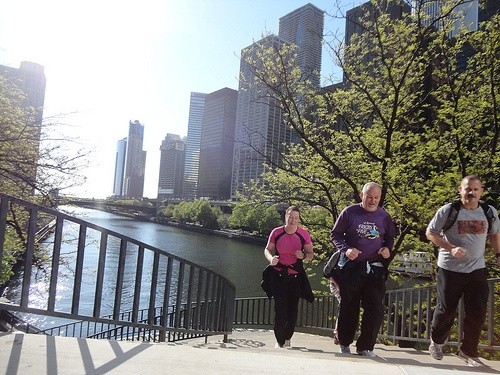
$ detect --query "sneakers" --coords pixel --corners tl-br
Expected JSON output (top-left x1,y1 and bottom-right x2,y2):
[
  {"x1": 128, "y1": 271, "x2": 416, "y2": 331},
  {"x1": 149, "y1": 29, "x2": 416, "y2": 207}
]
[
  {"x1": 457, "y1": 349, "x2": 483, "y2": 367},
  {"x1": 429, "y1": 336, "x2": 444, "y2": 360}
]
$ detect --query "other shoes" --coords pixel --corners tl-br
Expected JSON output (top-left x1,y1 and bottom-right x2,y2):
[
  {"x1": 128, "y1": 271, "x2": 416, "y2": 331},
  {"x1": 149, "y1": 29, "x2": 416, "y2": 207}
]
[
  {"x1": 334, "y1": 336, "x2": 339, "y2": 345},
  {"x1": 340, "y1": 345, "x2": 351, "y2": 354},
  {"x1": 284, "y1": 339, "x2": 290, "y2": 347},
  {"x1": 356, "y1": 349, "x2": 375, "y2": 358}
]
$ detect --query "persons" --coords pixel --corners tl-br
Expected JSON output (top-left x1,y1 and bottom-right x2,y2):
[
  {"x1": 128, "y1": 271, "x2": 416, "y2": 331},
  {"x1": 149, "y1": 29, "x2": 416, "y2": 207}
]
[
  {"x1": 323, "y1": 250, "x2": 341, "y2": 345},
  {"x1": 332, "y1": 181, "x2": 397, "y2": 357},
  {"x1": 425, "y1": 175, "x2": 500, "y2": 368},
  {"x1": 260, "y1": 205, "x2": 315, "y2": 349}
]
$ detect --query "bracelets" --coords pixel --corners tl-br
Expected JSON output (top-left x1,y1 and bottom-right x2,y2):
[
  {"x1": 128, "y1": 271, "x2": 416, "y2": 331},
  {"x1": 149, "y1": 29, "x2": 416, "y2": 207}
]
[
  {"x1": 496, "y1": 253, "x2": 500, "y2": 257},
  {"x1": 302, "y1": 251, "x2": 307, "y2": 260}
]
